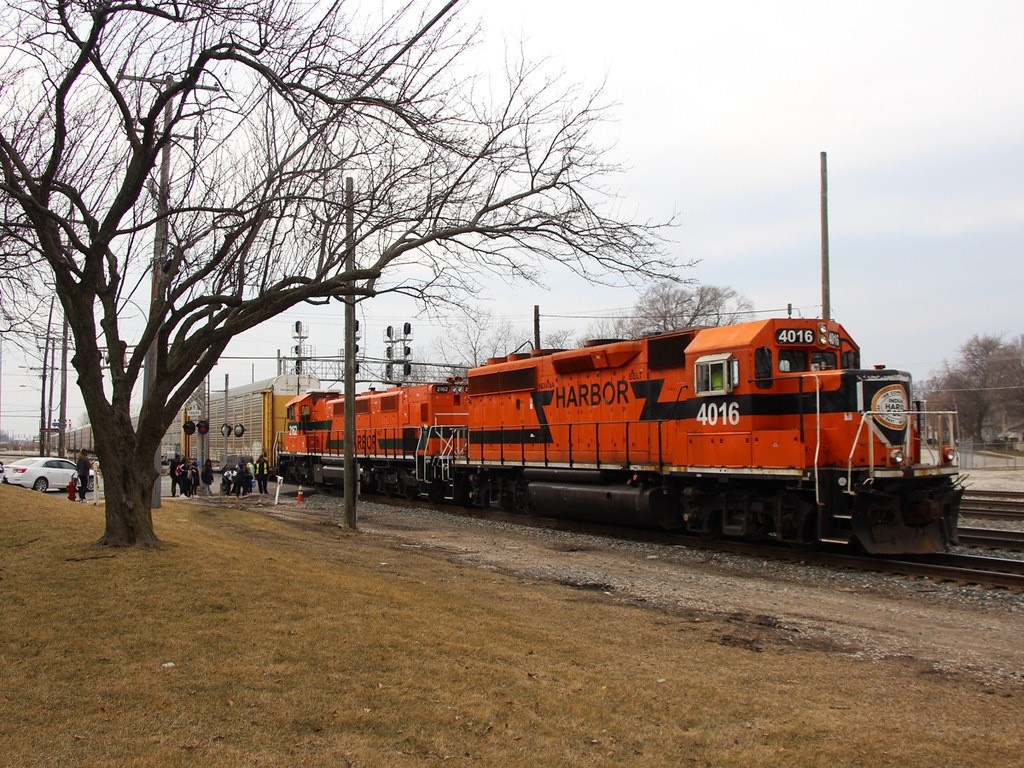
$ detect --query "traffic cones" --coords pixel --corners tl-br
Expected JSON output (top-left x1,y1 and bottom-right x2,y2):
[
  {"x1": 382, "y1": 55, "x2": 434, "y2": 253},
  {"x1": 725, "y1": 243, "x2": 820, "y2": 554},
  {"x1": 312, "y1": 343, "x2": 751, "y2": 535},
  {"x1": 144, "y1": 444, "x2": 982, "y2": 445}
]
[{"x1": 296, "y1": 485, "x2": 305, "y2": 508}]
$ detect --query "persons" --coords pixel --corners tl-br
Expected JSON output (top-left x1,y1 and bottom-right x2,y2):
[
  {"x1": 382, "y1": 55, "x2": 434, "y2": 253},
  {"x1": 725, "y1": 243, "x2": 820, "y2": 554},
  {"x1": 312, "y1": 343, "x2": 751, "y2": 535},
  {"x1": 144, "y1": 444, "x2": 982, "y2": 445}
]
[
  {"x1": 76, "y1": 448, "x2": 92, "y2": 502},
  {"x1": 221, "y1": 455, "x2": 255, "y2": 497},
  {"x1": 170, "y1": 455, "x2": 200, "y2": 498},
  {"x1": 201, "y1": 458, "x2": 214, "y2": 498},
  {"x1": 254, "y1": 455, "x2": 269, "y2": 494}
]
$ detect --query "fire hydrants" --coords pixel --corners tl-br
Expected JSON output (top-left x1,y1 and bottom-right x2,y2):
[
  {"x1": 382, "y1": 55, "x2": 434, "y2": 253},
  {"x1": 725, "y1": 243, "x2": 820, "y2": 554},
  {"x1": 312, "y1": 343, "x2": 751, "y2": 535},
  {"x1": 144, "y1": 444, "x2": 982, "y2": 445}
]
[{"x1": 67, "y1": 482, "x2": 77, "y2": 501}]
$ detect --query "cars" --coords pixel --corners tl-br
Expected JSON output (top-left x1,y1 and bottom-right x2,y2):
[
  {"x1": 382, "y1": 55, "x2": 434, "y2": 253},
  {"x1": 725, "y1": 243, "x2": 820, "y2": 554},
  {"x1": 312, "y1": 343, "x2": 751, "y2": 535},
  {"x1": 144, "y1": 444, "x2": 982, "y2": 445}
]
[{"x1": 0, "y1": 457, "x2": 98, "y2": 492}]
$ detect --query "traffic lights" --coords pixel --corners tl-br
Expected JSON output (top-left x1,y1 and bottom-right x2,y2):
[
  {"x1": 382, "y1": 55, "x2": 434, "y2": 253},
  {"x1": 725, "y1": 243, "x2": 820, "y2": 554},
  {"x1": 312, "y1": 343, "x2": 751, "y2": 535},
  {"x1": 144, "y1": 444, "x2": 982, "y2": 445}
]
[
  {"x1": 183, "y1": 421, "x2": 196, "y2": 435},
  {"x1": 196, "y1": 420, "x2": 209, "y2": 435}
]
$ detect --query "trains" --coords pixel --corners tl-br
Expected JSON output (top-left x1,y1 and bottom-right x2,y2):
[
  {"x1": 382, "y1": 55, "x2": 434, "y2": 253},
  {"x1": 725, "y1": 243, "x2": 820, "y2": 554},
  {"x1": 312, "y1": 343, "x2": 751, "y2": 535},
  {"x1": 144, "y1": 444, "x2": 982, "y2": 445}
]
[{"x1": 51, "y1": 317, "x2": 966, "y2": 562}]
